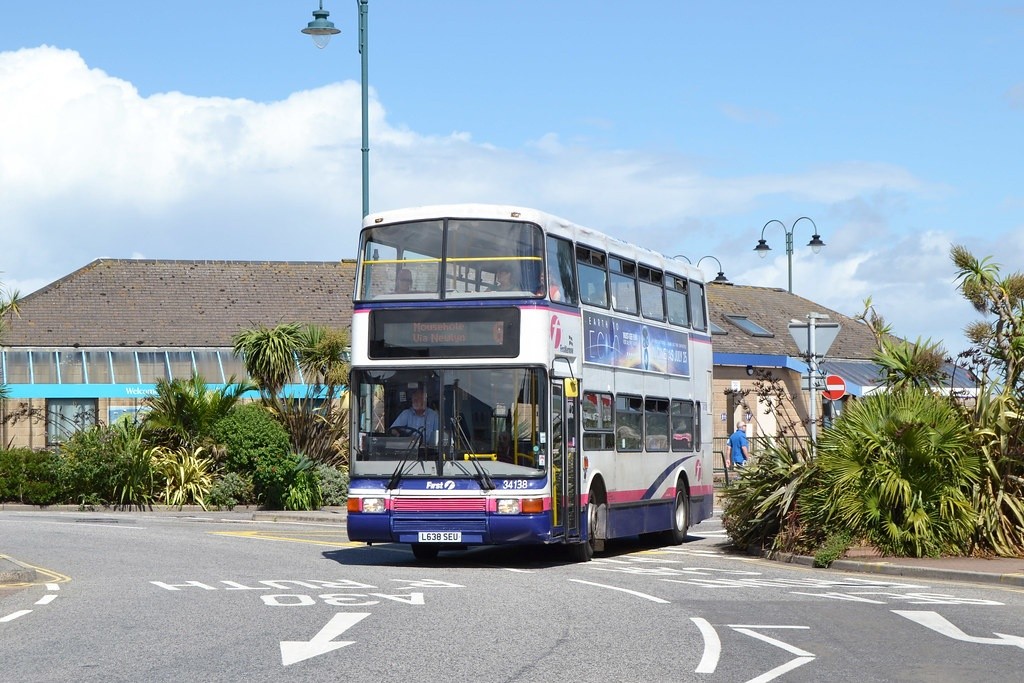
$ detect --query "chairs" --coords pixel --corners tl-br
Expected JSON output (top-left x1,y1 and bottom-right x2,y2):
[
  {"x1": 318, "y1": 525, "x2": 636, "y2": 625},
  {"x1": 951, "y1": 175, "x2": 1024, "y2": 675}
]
[{"x1": 646, "y1": 435, "x2": 668, "y2": 450}]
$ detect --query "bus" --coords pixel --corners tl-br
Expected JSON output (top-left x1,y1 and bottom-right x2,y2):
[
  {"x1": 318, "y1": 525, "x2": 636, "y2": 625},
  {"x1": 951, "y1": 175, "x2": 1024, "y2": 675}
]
[{"x1": 345, "y1": 203, "x2": 715, "y2": 563}]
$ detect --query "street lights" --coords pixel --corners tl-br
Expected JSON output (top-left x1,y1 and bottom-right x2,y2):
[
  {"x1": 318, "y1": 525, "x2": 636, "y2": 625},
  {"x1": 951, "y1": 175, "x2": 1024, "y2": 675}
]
[
  {"x1": 673, "y1": 255, "x2": 728, "y2": 282},
  {"x1": 301, "y1": 1, "x2": 370, "y2": 218},
  {"x1": 753, "y1": 217, "x2": 827, "y2": 295}
]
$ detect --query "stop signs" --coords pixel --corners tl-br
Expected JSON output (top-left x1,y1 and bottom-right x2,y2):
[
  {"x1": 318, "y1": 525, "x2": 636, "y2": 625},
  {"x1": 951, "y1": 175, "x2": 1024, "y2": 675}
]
[{"x1": 821, "y1": 376, "x2": 846, "y2": 401}]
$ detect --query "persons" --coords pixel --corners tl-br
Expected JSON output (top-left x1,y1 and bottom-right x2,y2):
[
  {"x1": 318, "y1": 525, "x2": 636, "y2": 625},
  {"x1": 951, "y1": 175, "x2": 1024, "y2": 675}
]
[
  {"x1": 726, "y1": 421, "x2": 749, "y2": 472},
  {"x1": 394, "y1": 269, "x2": 413, "y2": 293},
  {"x1": 535, "y1": 266, "x2": 561, "y2": 301},
  {"x1": 589, "y1": 276, "x2": 616, "y2": 309},
  {"x1": 485, "y1": 267, "x2": 521, "y2": 291},
  {"x1": 389, "y1": 389, "x2": 439, "y2": 447}
]
